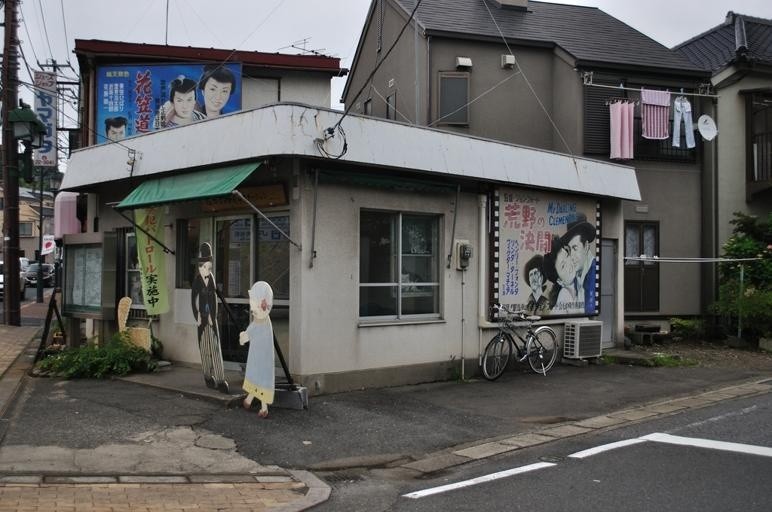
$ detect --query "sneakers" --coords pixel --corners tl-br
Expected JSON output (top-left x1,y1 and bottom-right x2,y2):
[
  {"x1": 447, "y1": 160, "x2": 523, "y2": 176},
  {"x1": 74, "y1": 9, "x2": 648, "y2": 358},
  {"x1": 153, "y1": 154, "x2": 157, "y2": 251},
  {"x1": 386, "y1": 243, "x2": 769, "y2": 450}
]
[
  {"x1": 242, "y1": 399, "x2": 250, "y2": 407},
  {"x1": 257, "y1": 411, "x2": 269, "y2": 417}
]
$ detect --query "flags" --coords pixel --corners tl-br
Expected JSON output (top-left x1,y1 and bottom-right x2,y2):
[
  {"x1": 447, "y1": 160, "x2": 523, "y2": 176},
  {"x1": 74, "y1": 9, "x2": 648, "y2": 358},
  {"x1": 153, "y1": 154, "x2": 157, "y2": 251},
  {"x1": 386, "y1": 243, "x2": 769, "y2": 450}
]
[{"x1": 42, "y1": 234, "x2": 55, "y2": 256}]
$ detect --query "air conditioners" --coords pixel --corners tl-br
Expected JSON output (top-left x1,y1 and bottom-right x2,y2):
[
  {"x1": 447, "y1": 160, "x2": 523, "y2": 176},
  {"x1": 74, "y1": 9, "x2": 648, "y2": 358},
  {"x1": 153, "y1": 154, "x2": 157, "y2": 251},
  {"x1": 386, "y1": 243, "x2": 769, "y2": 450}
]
[{"x1": 564, "y1": 320, "x2": 603, "y2": 360}]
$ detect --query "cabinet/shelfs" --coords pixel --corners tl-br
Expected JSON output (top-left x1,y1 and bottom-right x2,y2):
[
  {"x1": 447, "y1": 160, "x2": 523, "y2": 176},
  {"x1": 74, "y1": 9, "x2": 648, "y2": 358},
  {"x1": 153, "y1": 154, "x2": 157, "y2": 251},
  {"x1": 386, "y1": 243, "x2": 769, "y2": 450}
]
[{"x1": 389, "y1": 214, "x2": 434, "y2": 298}]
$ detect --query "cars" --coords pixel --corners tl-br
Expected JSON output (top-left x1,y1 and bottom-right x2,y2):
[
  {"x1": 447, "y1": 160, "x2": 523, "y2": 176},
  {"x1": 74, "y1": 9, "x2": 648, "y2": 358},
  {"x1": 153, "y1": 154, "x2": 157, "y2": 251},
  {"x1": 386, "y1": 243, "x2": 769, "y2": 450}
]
[
  {"x1": 0, "y1": 256, "x2": 27, "y2": 302},
  {"x1": 25, "y1": 263, "x2": 55, "y2": 288}
]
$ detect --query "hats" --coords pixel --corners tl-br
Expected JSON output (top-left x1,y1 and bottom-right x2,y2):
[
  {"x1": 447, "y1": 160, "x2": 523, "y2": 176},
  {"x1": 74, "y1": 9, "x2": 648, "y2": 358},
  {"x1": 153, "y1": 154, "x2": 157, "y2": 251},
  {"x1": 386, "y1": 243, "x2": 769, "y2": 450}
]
[
  {"x1": 559, "y1": 212, "x2": 596, "y2": 246},
  {"x1": 525, "y1": 255, "x2": 547, "y2": 285},
  {"x1": 192, "y1": 243, "x2": 213, "y2": 262}
]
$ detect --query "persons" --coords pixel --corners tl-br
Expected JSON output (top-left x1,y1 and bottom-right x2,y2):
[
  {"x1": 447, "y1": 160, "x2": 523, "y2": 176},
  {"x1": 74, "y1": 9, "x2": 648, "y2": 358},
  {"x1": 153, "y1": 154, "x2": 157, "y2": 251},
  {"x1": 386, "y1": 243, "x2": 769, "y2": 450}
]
[
  {"x1": 105, "y1": 116, "x2": 128, "y2": 142},
  {"x1": 524, "y1": 213, "x2": 596, "y2": 316},
  {"x1": 198, "y1": 64, "x2": 236, "y2": 117},
  {"x1": 164, "y1": 78, "x2": 207, "y2": 128}
]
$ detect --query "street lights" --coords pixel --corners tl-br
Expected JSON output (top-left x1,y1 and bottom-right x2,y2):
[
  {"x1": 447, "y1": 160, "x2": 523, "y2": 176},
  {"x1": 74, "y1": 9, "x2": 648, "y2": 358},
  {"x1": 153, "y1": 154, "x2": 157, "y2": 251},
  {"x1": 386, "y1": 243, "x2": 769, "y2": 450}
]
[{"x1": 2, "y1": 109, "x2": 47, "y2": 325}]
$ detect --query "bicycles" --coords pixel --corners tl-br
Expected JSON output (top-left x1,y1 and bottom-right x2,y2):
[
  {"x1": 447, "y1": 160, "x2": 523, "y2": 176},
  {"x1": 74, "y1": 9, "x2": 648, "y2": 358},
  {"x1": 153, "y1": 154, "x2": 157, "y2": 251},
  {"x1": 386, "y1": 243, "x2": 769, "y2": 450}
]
[{"x1": 482, "y1": 303, "x2": 559, "y2": 381}]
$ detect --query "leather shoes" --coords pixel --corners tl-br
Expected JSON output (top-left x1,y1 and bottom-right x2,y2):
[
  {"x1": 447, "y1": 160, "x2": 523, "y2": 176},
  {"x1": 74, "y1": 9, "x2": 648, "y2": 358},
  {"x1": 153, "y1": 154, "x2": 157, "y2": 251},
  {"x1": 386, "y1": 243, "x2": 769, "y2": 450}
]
[{"x1": 205, "y1": 376, "x2": 228, "y2": 393}]
